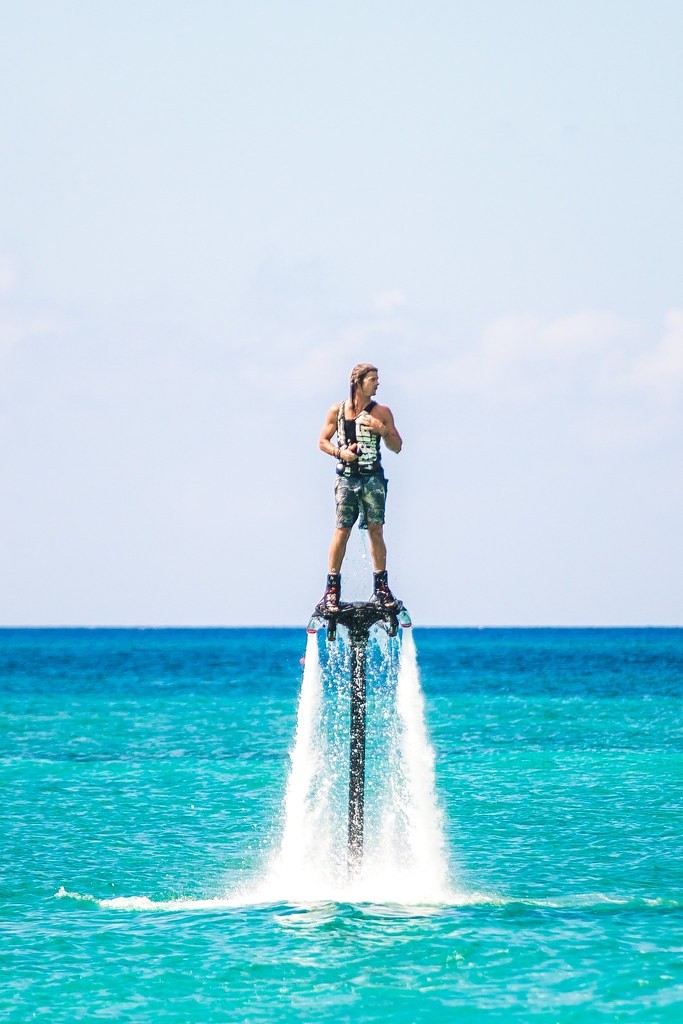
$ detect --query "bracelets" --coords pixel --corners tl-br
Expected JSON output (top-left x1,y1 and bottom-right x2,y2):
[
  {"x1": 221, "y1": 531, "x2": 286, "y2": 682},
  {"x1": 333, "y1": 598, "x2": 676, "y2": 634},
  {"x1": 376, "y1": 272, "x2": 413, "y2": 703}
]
[
  {"x1": 384, "y1": 429, "x2": 390, "y2": 437},
  {"x1": 332, "y1": 447, "x2": 341, "y2": 459}
]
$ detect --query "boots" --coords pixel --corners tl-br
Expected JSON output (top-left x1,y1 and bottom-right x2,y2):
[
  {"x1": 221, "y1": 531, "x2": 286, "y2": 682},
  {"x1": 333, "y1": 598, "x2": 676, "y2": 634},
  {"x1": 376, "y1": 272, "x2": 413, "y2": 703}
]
[
  {"x1": 324, "y1": 573, "x2": 343, "y2": 612},
  {"x1": 373, "y1": 571, "x2": 392, "y2": 602}
]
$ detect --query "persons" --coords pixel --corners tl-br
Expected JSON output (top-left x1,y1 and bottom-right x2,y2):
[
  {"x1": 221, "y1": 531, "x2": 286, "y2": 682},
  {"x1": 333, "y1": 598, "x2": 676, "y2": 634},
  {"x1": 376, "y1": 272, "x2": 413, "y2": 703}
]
[{"x1": 319, "y1": 362, "x2": 402, "y2": 609}]
